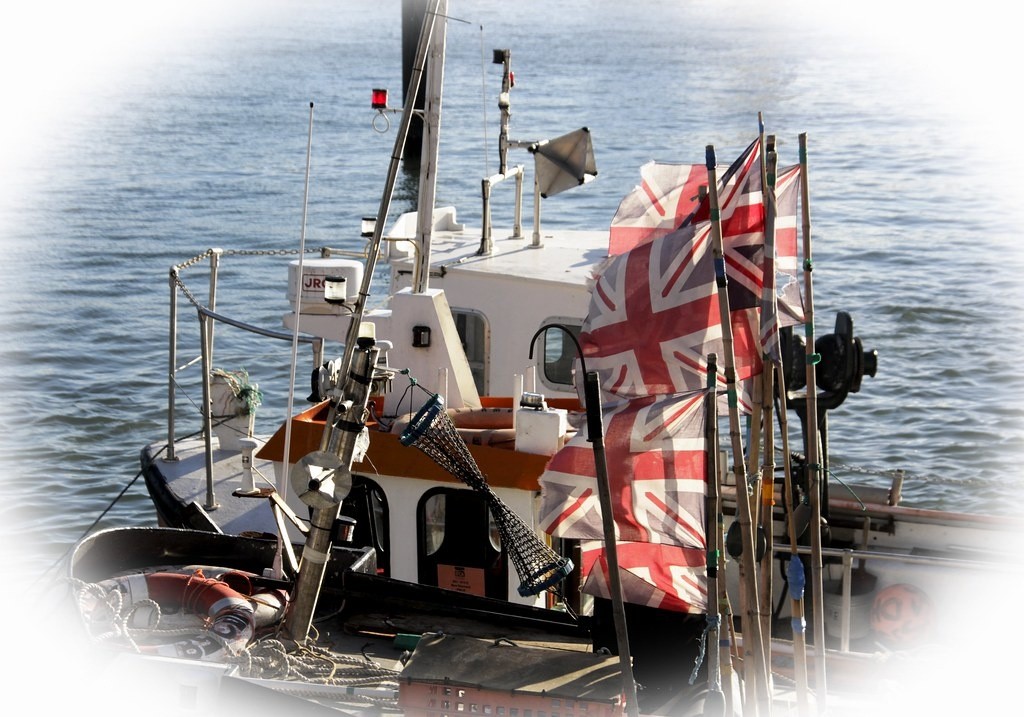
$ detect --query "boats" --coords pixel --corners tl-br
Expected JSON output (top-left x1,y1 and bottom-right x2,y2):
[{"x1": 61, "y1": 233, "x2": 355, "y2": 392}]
[{"x1": 53, "y1": 2, "x2": 998, "y2": 717}]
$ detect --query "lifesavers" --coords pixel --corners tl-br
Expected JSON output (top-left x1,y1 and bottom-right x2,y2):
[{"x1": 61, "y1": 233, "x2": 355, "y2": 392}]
[
  {"x1": 82, "y1": 570, "x2": 256, "y2": 662},
  {"x1": 102, "y1": 564, "x2": 291, "y2": 631}
]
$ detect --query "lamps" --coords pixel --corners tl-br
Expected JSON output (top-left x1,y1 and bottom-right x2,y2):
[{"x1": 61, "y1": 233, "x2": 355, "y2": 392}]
[
  {"x1": 360, "y1": 217, "x2": 419, "y2": 292},
  {"x1": 323, "y1": 274, "x2": 353, "y2": 313},
  {"x1": 411, "y1": 327, "x2": 431, "y2": 347},
  {"x1": 356, "y1": 322, "x2": 376, "y2": 350}
]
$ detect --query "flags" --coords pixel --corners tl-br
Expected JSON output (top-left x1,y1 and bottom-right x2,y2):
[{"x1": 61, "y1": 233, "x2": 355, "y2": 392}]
[{"x1": 538, "y1": 138, "x2": 804, "y2": 615}]
